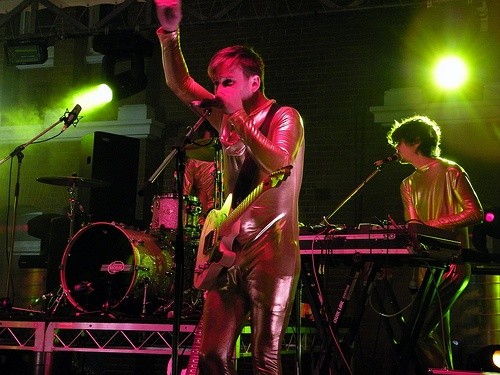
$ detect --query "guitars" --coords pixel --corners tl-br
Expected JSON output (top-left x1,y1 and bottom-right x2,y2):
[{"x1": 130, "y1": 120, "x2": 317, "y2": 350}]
[{"x1": 192, "y1": 163, "x2": 293, "y2": 294}]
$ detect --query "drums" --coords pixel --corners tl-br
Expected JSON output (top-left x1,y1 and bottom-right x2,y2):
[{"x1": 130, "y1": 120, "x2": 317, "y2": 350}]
[
  {"x1": 147, "y1": 189, "x2": 201, "y2": 239},
  {"x1": 56, "y1": 222, "x2": 177, "y2": 316}
]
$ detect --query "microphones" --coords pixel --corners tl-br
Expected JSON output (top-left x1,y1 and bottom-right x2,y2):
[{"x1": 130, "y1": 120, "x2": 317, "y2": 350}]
[
  {"x1": 191, "y1": 98, "x2": 223, "y2": 109},
  {"x1": 373, "y1": 152, "x2": 404, "y2": 167},
  {"x1": 62, "y1": 104, "x2": 82, "y2": 132}
]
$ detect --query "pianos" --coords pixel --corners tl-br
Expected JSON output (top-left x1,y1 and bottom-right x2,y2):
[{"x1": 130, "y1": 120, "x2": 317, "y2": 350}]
[{"x1": 298, "y1": 218, "x2": 472, "y2": 375}]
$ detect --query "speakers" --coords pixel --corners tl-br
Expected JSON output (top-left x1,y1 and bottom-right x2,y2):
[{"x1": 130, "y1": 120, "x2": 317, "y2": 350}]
[{"x1": 76, "y1": 131, "x2": 140, "y2": 219}]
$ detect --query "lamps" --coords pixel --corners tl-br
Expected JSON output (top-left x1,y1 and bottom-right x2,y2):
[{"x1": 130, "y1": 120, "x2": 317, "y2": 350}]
[
  {"x1": 91, "y1": 30, "x2": 153, "y2": 100},
  {"x1": 2, "y1": 37, "x2": 50, "y2": 67}
]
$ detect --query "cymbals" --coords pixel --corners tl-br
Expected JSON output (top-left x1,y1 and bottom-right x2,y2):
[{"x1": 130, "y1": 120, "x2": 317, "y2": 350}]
[{"x1": 35, "y1": 175, "x2": 105, "y2": 188}]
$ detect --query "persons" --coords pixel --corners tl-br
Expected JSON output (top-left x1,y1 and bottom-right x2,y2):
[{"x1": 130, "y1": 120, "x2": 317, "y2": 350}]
[
  {"x1": 386, "y1": 115, "x2": 483, "y2": 371},
  {"x1": 179, "y1": 127, "x2": 219, "y2": 225},
  {"x1": 156, "y1": 0, "x2": 305, "y2": 375}
]
